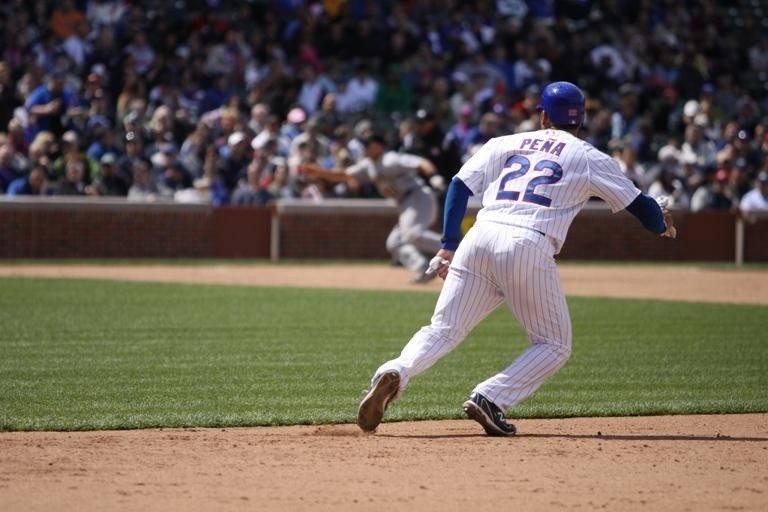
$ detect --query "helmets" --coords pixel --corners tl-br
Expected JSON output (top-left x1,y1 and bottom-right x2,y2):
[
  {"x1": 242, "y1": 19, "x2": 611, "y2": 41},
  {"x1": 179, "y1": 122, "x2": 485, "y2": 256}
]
[{"x1": 535, "y1": 81, "x2": 586, "y2": 128}]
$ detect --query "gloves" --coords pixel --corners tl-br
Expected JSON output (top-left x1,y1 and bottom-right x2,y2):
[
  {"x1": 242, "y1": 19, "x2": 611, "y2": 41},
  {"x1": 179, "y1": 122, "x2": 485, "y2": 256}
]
[{"x1": 426, "y1": 174, "x2": 448, "y2": 192}]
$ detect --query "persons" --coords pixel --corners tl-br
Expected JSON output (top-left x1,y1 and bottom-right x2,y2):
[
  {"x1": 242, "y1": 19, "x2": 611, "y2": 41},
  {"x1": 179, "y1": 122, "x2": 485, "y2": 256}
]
[
  {"x1": 300, "y1": 128, "x2": 446, "y2": 283},
  {"x1": 358, "y1": 81, "x2": 677, "y2": 436},
  {"x1": 1, "y1": 0, "x2": 768, "y2": 213}
]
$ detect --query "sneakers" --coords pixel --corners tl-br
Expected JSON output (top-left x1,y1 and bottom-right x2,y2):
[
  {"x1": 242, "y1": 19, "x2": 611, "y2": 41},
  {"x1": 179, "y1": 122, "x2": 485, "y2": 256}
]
[
  {"x1": 406, "y1": 269, "x2": 437, "y2": 284},
  {"x1": 461, "y1": 391, "x2": 517, "y2": 437},
  {"x1": 356, "y1": 368, "x2": 401, "y2": 433}
]
